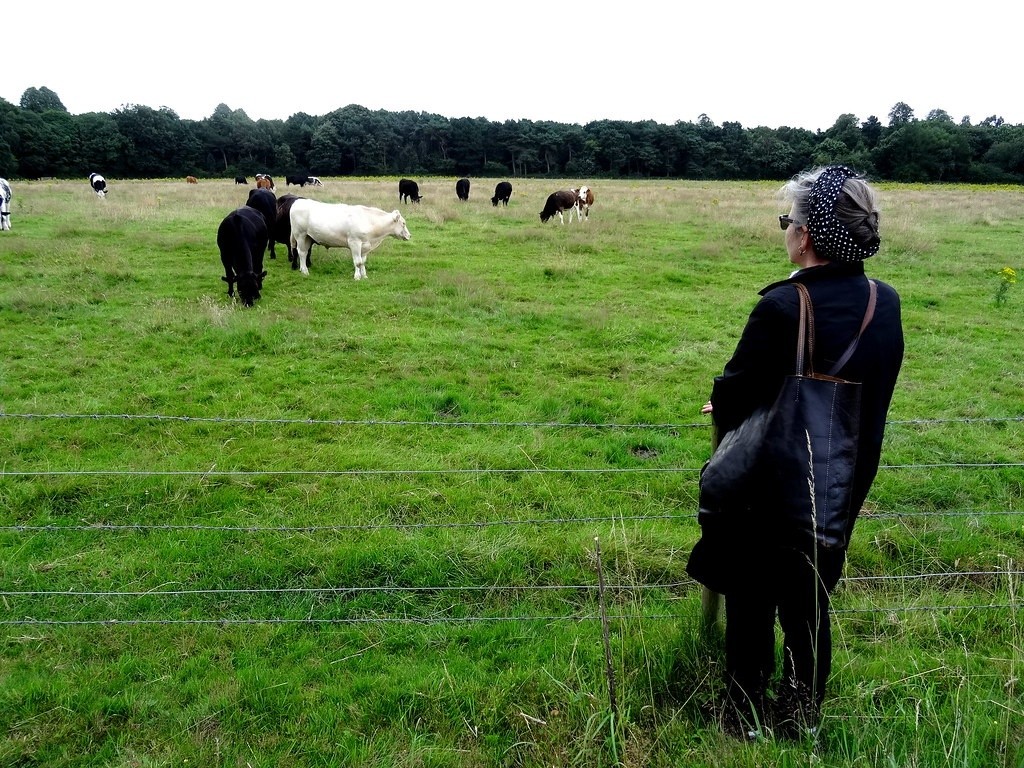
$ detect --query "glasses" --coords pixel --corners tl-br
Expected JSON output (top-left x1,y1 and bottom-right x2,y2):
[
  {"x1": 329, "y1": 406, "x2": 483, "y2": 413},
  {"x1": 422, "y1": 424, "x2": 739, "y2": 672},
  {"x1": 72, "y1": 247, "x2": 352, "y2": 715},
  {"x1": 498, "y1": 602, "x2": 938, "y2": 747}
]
[{"x1": 779, "y1": 214, "x2": 807, "y2": 230}]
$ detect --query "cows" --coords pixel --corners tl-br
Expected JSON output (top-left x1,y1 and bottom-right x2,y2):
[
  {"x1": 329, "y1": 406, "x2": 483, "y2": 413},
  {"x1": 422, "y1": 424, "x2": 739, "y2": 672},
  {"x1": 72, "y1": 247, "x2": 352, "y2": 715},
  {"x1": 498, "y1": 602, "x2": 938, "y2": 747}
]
[
  {"x1": 0, "y1": 177, "x2": 12, "y2": 231},
  {"x1": 186, "y1": 176, "x2": 197, "y2": 184},
  {"x1": 539, "y1": 186, "x2": 594, "y2": 224},
  {"x1": 89, "y1": 173, "x2": 108, "y2": 199},
  {"x1": 399, "y1": 179, "x2": 423, "y2": 205},
  {"x1": 456, "y1": 179, "x2": 470, "y2": 201},
  {"x1": 217, "y1": 174, "x2": 411, "y2": 307},
  {"x1": 491, "y1": 182, "x2": 512, "y2": 207}
]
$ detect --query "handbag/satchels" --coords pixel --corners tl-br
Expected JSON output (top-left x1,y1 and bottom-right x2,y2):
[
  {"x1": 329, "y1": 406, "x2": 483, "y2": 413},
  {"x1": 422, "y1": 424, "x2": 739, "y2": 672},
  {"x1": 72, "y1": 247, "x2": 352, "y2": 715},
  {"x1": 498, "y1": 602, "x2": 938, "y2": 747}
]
[{"x1": 697, "y1": 279, "x2": 860, "y2": 550}]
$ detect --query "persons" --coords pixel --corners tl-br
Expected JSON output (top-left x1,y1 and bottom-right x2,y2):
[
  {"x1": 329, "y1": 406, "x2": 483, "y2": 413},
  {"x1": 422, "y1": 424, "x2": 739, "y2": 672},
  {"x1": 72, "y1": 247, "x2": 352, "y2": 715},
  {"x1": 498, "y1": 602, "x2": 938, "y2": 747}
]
[{"x1": 686, "y1": 164, "x2": 904, "y2": 743}]
